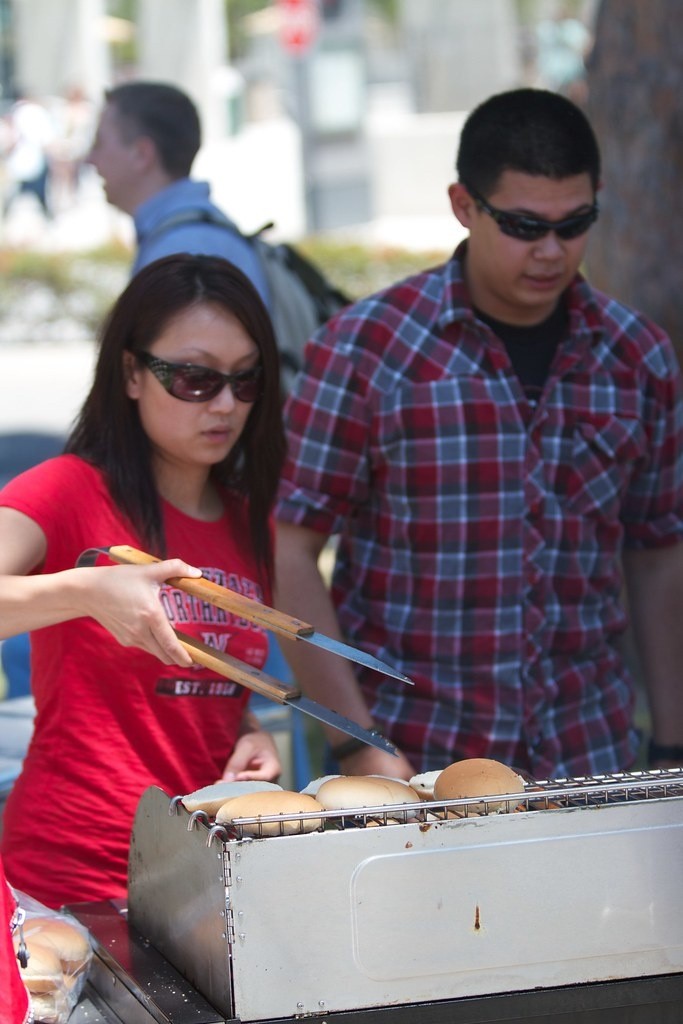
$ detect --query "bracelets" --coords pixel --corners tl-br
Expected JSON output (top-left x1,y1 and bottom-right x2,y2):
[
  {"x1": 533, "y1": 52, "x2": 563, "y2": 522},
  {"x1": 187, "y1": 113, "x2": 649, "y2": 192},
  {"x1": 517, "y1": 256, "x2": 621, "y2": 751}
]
[{"x1": 333, "y1": 724, "x2": 384, "y2": 759}]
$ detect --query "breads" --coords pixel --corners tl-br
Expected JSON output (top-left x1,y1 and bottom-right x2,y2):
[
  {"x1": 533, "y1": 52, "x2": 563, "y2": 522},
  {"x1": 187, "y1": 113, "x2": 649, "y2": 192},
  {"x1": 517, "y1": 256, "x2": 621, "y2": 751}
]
[
  {"x1": 180, "y1": 758, "x2": 526, "y2": 837},
  {"x1": 11, "y1": 913, "x2": 91, "y2": 1024}
]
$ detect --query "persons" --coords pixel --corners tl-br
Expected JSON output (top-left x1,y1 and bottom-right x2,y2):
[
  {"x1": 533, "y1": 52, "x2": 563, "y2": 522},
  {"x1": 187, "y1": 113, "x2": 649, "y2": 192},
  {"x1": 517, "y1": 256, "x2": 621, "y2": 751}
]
[
  {"x1": 0, "y1": 88, "x2": 88, "y2": 221},
  {"x1": 0, "y1": 252, "x2": 285, "y2": 923},
  {"x1": 88, "y1": 82, "x2": 271, "y2": 319},
  {"x1": 271, "y1": 88, "x2": 683, "y2": 785}
]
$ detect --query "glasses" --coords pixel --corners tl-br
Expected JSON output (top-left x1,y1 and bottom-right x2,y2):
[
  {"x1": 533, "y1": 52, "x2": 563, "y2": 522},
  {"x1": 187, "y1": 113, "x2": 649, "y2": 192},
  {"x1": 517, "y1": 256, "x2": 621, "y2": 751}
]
[
  {"x1": 461, "y1": 182, "x2": 601, "y2": 240},
  {"x1": 129, "y1": 348, "x2": 268, "y2": 404}
]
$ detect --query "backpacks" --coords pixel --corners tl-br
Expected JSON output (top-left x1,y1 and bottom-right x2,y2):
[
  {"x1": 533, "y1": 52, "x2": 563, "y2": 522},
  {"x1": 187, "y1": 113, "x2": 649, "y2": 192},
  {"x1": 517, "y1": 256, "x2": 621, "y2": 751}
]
[{"x1": 144, "y1": 210, "x2": 359, "y2": 405}]
